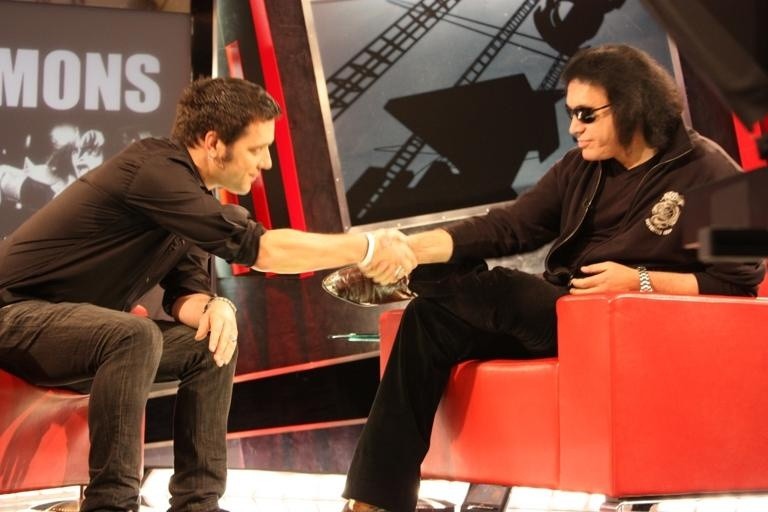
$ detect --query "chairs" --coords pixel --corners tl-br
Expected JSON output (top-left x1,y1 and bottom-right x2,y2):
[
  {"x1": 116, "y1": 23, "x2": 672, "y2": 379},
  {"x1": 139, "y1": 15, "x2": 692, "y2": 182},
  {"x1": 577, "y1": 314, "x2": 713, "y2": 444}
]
[{"x1": 371, "y1": 275, "x2": 767, "y2": 511}]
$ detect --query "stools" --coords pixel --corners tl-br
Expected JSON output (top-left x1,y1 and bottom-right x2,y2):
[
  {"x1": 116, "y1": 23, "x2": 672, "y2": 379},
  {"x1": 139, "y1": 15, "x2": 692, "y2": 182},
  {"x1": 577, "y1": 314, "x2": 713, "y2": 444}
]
[{"x1": 1, "y1": 372, "x2": 151, "y2": 512}]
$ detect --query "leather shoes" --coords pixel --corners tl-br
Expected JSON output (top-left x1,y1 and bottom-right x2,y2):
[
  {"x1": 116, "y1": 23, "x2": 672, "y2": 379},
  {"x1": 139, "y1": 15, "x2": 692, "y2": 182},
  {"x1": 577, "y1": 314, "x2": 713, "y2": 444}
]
[{"x1": 321, "y1": 265, "x2": 418, "y2": 307}]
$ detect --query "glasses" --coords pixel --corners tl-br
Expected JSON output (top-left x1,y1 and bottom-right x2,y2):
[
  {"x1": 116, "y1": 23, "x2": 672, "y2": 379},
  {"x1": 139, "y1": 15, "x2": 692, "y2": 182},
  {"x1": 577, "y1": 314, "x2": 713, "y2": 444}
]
[{"x1": 563, "y1": 101, "x2": 613, "y2": 124}]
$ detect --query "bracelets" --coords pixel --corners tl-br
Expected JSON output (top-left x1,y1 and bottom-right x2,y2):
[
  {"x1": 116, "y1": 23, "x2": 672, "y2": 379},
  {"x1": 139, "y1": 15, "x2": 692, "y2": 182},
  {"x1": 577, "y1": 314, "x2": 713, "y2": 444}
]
[
  {"x1": 359, "y1": 232, "x2": 376, "y2": 267},
  {"x1": 206, "y1": 296, "x2": 240, "y2": 318},
  {"x1": 637, "y1": 266, "x2": 653, "y2": 295}
]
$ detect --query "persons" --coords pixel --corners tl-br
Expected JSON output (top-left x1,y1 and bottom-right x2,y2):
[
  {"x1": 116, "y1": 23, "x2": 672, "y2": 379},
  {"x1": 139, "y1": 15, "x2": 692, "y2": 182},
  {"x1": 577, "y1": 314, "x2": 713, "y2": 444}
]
[
  {"x1": 1, "y1": 77, "x2": 417, "y2": 512},
  {"x1": 342, "y1": 43, "x2": 763, "y2": 511}
]
